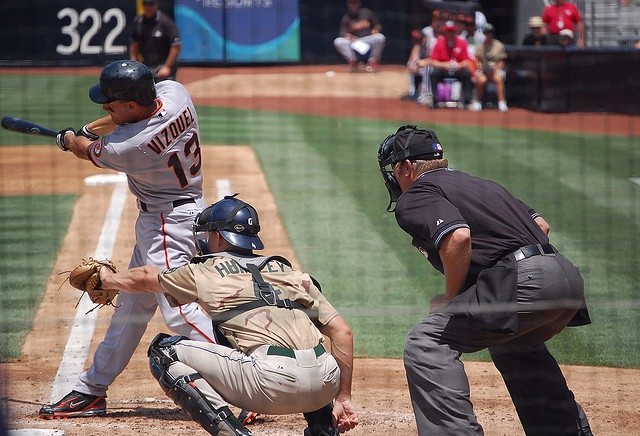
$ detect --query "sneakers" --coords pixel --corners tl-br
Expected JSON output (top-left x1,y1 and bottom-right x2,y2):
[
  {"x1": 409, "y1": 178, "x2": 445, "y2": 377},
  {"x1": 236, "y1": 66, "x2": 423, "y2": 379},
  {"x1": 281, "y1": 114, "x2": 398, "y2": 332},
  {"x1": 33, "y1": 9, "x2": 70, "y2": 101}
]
[
  {"x1": 345, "y1": 61, "x2": 359, "y2": 72},
  {"x1": 498, "y1": 100, "x2": 508, "y2": 112},
  {"x1": 466, "y1": 99, "x2": 482, "y2": 111},
  {"x1": 39, "y1": 390, "x2": 107, "y2": 420},
  {"x1": 304, "y1": 425, "x2": 342, "y2": 436},
  {"x1": 401, "y1": 94, "x2": 418, "y2": 100},
  {"x1": 365, "y1": 60, "x2": 378, "y2": 73},
  {"x1": 238, "y1": 407, "x2": 258, "y2": 426}
]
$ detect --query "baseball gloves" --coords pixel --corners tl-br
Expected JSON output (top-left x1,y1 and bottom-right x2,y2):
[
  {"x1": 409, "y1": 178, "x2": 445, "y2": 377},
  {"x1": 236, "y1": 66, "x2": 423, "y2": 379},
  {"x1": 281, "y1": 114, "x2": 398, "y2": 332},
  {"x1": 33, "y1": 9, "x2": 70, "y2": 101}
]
[{"x1": 57, "y1": 256, "x2": 122, "y2": 314}]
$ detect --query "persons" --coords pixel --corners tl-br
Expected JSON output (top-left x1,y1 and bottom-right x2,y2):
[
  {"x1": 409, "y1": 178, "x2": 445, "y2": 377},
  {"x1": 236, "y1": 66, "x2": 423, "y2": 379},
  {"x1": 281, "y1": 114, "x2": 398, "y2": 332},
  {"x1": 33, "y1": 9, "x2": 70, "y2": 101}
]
[
  {"x1": 334, "y1": 2, "x2": 385, "y2": 73},
  {"x1": 558, "y1": 29, "x2": 577, "y2": 48},
  {"x1": 407, "y1": 12, "x2": 510, "y2": 113},
  {"x1": 88, "y1": 195, "x2": 358, "y2": 436},
  {"x1": 378, "y1": 126, "x2": 595, "y2": 434},
  {"x1": 39, "y1": 60, "x2": 258, "y2": 425},
  {"x1": 129, "y1": 0, "x2": 180, "y2": 82},
  {"x1": 522, "y1": 17, "x2": 552, "y2": 44},
  {"x1": 614, "y1": 0, "x2": 640, "y2": 49},
  {"x1": 543, "y1": 0, "x2": 583, "y2": 47}
]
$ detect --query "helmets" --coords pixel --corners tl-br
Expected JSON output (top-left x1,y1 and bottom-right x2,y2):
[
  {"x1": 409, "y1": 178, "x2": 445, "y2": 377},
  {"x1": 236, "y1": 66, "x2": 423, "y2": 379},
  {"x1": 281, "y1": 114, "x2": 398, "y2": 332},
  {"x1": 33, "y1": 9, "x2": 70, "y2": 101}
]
[
  {"x1": 378, "y1": 126, "x2": 443, "y2": 212},
  {"x1": 442, "y1": 21, "x2": 456, "y2": 34},
  {"x1": 89, "y1": 60, "x2": 156, "y2": 106},
  {"x1": 192, "y1": 196, "x2": 264, "y2": 255},
  {"x1": 484, "y1": 24, "x2": 495, "y2": 34}
]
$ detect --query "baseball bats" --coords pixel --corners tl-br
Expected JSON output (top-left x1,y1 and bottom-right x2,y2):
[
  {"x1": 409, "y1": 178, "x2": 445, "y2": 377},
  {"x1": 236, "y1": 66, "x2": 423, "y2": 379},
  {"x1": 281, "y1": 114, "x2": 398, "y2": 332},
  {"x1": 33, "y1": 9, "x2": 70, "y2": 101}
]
[{"x1": 1, "y1": 114, "x2": 58, "y2": 138}]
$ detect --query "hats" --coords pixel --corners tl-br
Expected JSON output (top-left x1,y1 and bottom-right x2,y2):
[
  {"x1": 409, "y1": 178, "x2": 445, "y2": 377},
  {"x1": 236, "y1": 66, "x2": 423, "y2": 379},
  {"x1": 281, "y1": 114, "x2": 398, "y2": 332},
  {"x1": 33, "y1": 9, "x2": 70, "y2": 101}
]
[
  {"x1": 409, "y1": 30, "x2": 424, "y2": 41},
  {"x1": 560, "y1": 29, "x2": 574, "y2": 39},
  {"x1": 528, "y1": 16, "x2": 544, "y2": 28}
]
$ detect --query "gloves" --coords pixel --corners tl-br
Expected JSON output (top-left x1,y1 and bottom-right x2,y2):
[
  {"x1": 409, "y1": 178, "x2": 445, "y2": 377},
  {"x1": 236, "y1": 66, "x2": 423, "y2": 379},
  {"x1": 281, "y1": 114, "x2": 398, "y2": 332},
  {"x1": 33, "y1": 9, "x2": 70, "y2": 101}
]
[
  {"x1": 57, "y1": 127, "x2": 76, "y2": 151},
  {"x1": 76, "y1": 123, "x2": 100, "y2": 141}
]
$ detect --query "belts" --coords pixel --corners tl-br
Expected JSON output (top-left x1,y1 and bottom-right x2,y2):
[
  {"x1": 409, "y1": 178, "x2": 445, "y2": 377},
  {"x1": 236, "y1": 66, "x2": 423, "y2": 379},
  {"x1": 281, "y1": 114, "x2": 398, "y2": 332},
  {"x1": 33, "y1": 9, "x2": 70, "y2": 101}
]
[
  {"x1": 267, "y1": 343, "x2": 326, "y2": 358},
  {"x1": 140, "y1": 197, "x2": 196, "y2": 212},
  {"x1": 514, "y1": 244, "x2": 559, "y2": 262}
]
